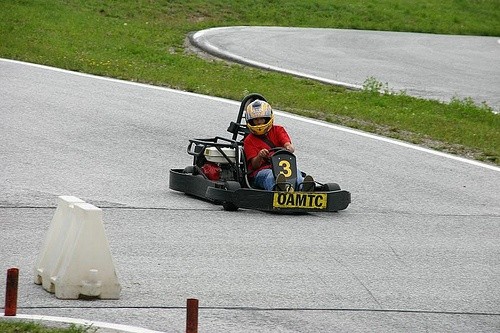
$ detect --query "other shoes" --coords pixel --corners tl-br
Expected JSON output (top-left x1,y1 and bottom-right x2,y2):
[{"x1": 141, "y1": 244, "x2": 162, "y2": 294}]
[
  {"x1": 276, "y1": 174, "x2": 288, "y2": 191},
  {"x1": 300, "y1": 175, "x2": 315, "y2": 192}
]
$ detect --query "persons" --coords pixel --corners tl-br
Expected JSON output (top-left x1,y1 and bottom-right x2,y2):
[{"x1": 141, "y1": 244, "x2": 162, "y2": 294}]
[{"x1": 245, "y1": 99, "x2": 304, "y2": 190}]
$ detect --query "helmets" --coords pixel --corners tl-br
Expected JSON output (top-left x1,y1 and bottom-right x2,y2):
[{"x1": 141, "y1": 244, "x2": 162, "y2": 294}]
[{"x1": 245, "y1": 99, "x2": 274, "y2": 135}]
